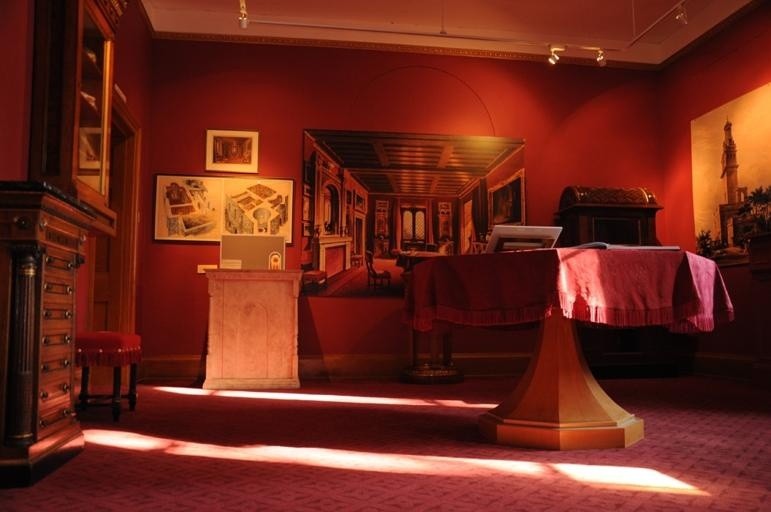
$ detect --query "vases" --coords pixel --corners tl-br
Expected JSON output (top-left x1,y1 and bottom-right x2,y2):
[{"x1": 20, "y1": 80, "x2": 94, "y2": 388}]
[{"x1": 745, "y1": 230, "x2": 771, "y2": 273}]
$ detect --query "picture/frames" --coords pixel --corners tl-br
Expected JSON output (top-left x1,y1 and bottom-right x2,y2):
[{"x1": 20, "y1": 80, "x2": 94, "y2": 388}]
[
  {"x1": 152, "y1": 127, "x2": 295, "y2": 248},
  {"x1": 487, "y1": 168, "x2": 526, "y2": 230},
  {"x1": 304, "y1": 162, "x2": 312, "y2": 236}
]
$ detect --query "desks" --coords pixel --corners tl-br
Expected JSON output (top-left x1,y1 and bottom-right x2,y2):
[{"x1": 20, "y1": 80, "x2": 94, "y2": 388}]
[{"x1": 402, "y1": 248, "x2": 719, "y2": 449}]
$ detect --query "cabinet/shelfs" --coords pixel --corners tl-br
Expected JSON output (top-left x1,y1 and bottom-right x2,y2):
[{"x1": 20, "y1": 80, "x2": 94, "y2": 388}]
[{"x1": 29, "y1": 0, "x2": 114, "y2": 207}]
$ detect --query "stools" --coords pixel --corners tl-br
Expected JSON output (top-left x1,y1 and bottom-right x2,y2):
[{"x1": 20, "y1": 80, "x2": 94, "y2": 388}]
[
  {"x1": 76, "y1": 331, "x2": 144, "y2": 421},
  {"x1": 351, "y1": 253, "x2": 363, "y2": 270},
  {"x1": 303, "y1": 271, "x2": 328, "y2": 293}
]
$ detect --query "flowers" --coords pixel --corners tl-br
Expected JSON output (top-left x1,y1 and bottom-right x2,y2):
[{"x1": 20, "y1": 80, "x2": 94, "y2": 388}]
[{"x1": 737, "y1": 186, "x2": 771, "y2": 240}]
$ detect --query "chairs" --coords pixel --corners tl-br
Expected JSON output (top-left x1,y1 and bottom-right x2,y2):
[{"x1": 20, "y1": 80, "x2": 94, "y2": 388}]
[{"x1": 364, "y1": 250, "x2": 391, "y2": 292}]
